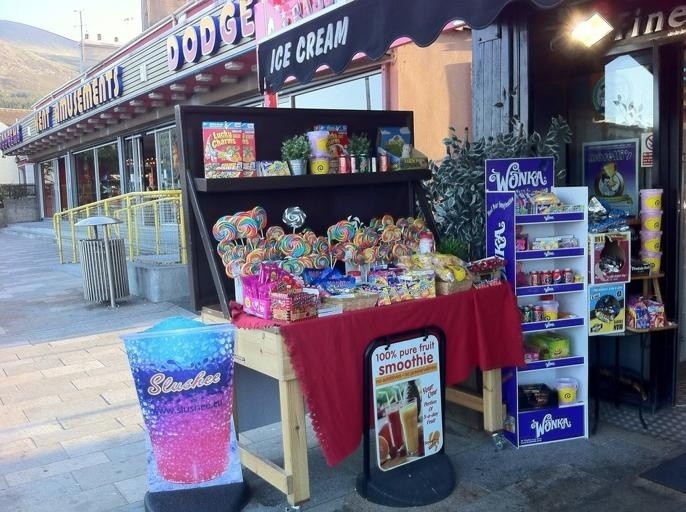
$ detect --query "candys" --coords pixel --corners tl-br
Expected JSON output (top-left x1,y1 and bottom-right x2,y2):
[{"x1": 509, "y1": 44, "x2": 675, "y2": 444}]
[{"x1": 212, "y1": 204, "x2": 426, "y2": 278}]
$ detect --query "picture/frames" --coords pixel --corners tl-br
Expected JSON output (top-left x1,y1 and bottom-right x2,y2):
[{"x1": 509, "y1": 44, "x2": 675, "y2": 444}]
[{"x1": 582, "y1": 139, "x2": 640, "y2": 227}]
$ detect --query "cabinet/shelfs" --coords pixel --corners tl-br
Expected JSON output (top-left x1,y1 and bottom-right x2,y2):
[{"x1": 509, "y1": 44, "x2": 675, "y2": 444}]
[
  {"x1": 587, "y1": 190, "x2": 679, "y2": 432},
  {"x1": 167, "y1": 101, "x2": 514, "y2": 506},
  {"x1": 484, "y1": 156, "x2": 594, "y2": 450}
]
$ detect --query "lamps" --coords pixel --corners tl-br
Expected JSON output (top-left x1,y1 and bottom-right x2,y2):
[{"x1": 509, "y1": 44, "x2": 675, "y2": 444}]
[{"x1": 566, "y1": 1, "x2": 618, "y2": 55}]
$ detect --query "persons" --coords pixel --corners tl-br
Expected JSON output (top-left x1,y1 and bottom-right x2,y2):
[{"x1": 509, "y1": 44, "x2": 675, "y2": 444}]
[{"x1": 601, "y1": 172, "x2": 620, "y2": 196}]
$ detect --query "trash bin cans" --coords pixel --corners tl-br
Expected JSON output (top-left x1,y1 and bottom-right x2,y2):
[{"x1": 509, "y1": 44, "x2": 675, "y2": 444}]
[{"x1": 74, "y1": 216, "x2": 130, "y2": 309}]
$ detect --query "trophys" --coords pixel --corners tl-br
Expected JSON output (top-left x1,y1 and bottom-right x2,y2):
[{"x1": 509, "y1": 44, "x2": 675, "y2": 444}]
[{"x1": 600, "y1": 161, "x2": 618, "y2": 187}]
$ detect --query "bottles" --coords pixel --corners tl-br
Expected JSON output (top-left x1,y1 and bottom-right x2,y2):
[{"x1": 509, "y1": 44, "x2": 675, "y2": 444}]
[
  {"x1": 537, "y1": 295, "x2": 560, "y2": 321},
  {"x1": 636, "y1": 187, "x2": 665, "y2": 273}
]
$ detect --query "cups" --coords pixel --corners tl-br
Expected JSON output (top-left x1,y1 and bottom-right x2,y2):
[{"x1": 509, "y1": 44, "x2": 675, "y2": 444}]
[
  {"x1": 554, "y1": 377, "x2": 578, "y2": 405},
  {"x1": 118, "y1": 322, "x2": 244, "y2": 484},
  {"x1": 383, "y1": 395, "x2": 422, "y2": 457}
]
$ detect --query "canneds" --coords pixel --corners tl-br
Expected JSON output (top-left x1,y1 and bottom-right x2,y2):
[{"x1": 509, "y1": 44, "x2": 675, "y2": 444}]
[
  {"x1": 338, "y1": 153, "x2": 390, "y2": 174},
  {"x1": 528, "y1": 269, "x2": 573, "y2": 286},
  {"x1": 518, "y1": 304, "x2": 543, "y2": 323}
]
